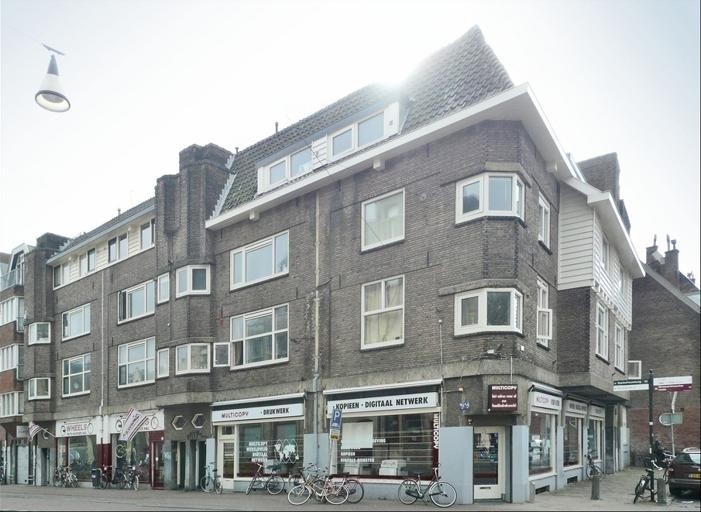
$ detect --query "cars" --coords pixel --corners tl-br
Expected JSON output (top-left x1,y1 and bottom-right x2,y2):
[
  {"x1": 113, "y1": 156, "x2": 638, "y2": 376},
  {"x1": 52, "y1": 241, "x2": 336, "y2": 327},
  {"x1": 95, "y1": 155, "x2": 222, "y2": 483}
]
[{"x1": 668, "y1": 447, "x2": 701, "y2": 496}]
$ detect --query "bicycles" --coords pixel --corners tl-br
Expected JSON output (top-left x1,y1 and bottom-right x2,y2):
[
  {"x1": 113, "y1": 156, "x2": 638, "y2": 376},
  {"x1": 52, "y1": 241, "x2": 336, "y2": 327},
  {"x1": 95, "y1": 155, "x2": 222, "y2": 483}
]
[
  {"x1": 52, "y1": 464, "x2": 78, "y2": 488},
  {"x1": 634, "y1": 452, "x2": 677, "y2": 504},
  {"x1": 288, "y1": 462, "x2": 364, "y2": 505},
  {"x1": 246, "y1": 461, "x2": 285, "y2": 495},
  {"x1": 99, "y1": 464, "x2": 141, "y2": 490},
  {"x1": 584, "y1": 450, "x2": 604, "y2": 481},
  {"x1": 200, "y1": 462, "x2": 223, "y2": 495},
  {"x1": 397, "y1": 463, "x2": 457, "y2": 508}
]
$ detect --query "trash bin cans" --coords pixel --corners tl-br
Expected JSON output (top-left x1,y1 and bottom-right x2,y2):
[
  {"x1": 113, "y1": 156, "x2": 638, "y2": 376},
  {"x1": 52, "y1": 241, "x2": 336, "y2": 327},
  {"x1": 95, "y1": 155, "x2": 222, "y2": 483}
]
[{"x1": 91, "y1": 469, "x2": 100, "y2": 487}]
